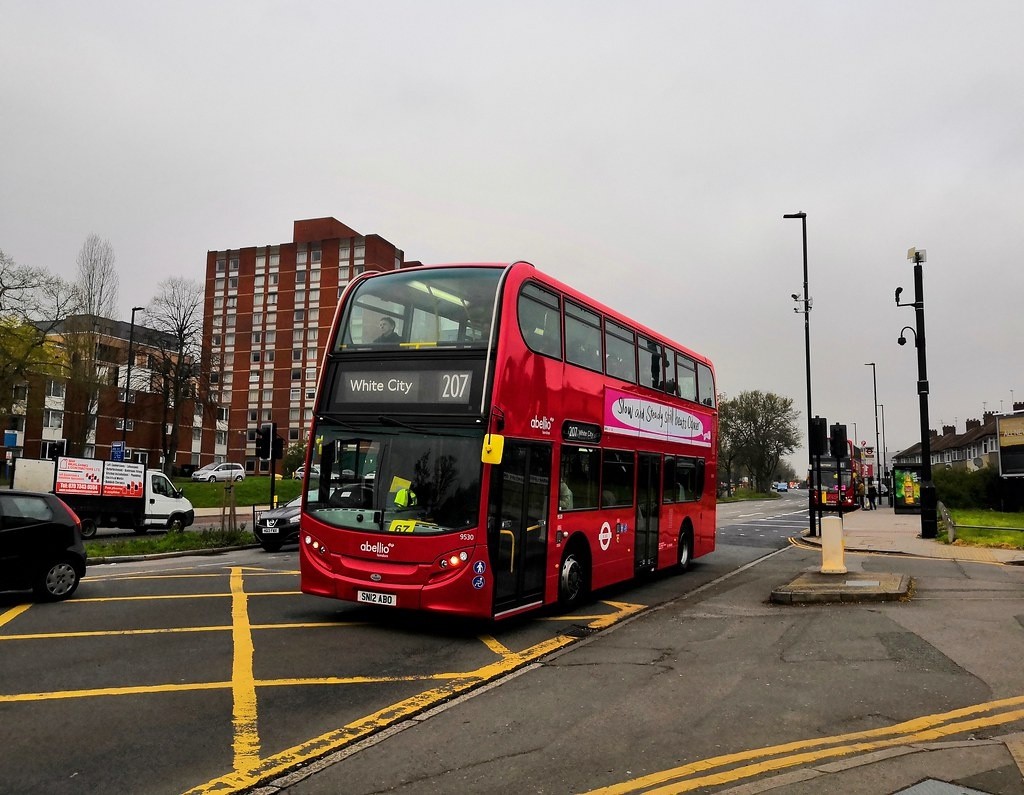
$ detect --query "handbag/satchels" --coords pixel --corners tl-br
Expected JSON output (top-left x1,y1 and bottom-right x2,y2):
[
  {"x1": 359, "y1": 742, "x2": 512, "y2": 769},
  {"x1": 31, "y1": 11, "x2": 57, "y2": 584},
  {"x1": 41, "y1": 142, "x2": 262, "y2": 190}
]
[
  {"x1": 865, "y1": 498, "x2": 870, "y2": 509},
  {"x1": 874, "y1": 497, "x2": 877, "y2": 505}
]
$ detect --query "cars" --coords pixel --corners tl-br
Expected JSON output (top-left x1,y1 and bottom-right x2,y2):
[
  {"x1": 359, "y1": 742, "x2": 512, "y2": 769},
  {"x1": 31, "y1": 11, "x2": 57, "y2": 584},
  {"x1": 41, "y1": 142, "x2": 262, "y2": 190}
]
[
  {"x1": 293, "y1": 465, "x2": 376, "y2": 481},
  {"x1": 771, "y1": 482, "x2": 780, "y2": 490},
  {"x1": 777, "y1": 483, "x2": 788, "y2": 492},
  {"x1": 254, "y1": 487, "x2": 353, "y2": 552},
  {"x1": 0, "y1": 488, "x2": 86, "y2": 601},
  {"x1": 191, "y1": 462, "x2": 246, "y2": 483}
]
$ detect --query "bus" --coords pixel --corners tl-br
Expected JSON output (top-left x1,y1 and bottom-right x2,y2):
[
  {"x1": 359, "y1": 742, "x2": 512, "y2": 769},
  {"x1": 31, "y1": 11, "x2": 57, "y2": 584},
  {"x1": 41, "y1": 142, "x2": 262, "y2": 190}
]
[
  {"x1": 297, "y1": 260, "x2": 720, "y2": 620},
  {"x1": 807, "y1": 438, "x2": 865, "y2": 512}
]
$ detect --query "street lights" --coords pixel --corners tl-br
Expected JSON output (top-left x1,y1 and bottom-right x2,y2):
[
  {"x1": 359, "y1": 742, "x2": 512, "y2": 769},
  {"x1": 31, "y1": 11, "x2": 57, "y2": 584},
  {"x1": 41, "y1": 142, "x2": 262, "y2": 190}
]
[
  {"x1": 877, "y1": 404, "x2": 886, "y2": 484},
  {"x1": 895, "y1": 246, "x2": 938, "y2": 539},
  {"x1": 121, "y1": 306, "x2": 145, "y2": 463},
  {"x1": 782, "y1": 211, "x2": 818, "y2": 537},
  {"x1": 864, "y1": 362, "x2": 882, "y2": 506},
  {"x1": 851, "y1": 422, "x2": 857, "y2": 445}
]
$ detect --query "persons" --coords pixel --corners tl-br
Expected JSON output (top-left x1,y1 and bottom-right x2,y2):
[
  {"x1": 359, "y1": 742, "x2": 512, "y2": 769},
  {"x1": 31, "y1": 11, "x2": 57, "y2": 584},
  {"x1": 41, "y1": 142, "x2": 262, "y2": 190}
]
[
  {"x1": 868, "y1": 481, "x2": 876, "y2": 510},
  {"x1": 854, "y1": 480, "x2": 865, "y2": 510},
  {"x1": 372, "y1": 317, "x2": 404, "y2": 351},
  {"x1": 602, "y1": 490, "x2": 617, "y2": 506},
  {"x1": 422, "y1": 455, "x2": 464, "y2": 527},
  {"x1": 471, "y1": 320, "x2": 491, "y2": 347},
  {"x1": 559, "y1": 476, "x2": 573, "y2": 510}
]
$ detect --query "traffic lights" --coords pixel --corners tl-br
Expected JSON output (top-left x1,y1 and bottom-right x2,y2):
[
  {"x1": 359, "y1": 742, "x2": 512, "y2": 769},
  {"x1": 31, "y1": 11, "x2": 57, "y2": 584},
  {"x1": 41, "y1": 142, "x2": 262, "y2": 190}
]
[
  {"x1": 254, "y1": 423, "x2": 272, "y2": 461},
  {"x1": 46, "y1": 442, "x2": 61, "y2": 460}
]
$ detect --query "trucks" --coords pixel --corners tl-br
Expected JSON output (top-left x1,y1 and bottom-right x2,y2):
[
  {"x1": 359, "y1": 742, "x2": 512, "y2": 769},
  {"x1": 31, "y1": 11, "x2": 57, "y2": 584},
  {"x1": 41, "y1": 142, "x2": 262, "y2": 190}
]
[{"x1": 8, "y1": 455, "x2": 195, "y2": 541}]
